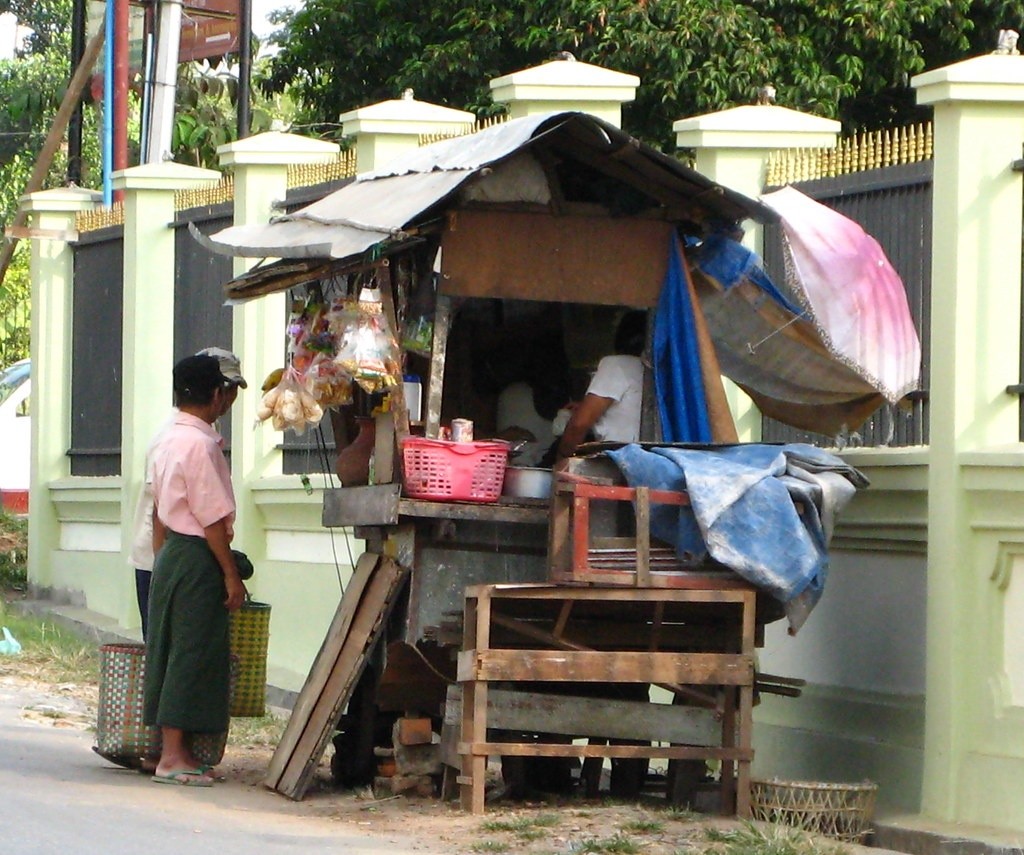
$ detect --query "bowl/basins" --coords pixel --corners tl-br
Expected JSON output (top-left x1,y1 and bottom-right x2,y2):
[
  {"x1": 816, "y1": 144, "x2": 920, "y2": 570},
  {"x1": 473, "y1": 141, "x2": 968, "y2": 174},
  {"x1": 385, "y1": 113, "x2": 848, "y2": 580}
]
[{"x1": 503, "y1": 465, "x2": 553, "y2": 498}]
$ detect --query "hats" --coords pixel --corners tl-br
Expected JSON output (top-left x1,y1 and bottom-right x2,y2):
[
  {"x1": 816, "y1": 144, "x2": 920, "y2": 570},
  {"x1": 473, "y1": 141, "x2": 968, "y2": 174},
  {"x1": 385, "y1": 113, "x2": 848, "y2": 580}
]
[
  {"x1": 174, "y1": 356, "x2": 232, "y2": 390},
  {"x1": 196, "y1": 347, "x2": 248, "y2": 389}
]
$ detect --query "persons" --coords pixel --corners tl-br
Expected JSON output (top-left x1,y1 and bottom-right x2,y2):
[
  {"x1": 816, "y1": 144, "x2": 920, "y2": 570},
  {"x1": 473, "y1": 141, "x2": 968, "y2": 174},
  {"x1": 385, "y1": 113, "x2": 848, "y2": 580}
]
[
  {"x1": 555, "y1": 311, "x2": 646, "y2": 460},
  {"x1": 129, "y1": 347, "x2": 251, "y2": 785}
]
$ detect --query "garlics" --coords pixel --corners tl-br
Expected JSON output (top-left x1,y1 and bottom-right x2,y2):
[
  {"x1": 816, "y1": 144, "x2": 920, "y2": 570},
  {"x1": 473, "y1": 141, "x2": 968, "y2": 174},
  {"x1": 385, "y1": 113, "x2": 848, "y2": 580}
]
[{"x1": 257, "y1": 388, "x2": 323, "y2": 429}]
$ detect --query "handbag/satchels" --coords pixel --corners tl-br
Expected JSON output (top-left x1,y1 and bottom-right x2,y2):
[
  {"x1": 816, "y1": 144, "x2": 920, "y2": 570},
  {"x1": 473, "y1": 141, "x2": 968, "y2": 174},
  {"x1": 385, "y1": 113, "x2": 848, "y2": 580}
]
[
  {"x1": 92, "y1": 644, "x2": 238, "y2": 767},
  {"x1": 227, "y1": 579, "x2": 270, "y2": 717}
]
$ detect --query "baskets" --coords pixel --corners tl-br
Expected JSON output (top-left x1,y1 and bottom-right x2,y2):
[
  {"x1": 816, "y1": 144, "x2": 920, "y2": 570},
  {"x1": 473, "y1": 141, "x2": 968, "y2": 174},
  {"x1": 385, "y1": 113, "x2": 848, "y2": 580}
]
[{"x1": 402, "y1": 434, "x2": 513, "y2": 501}]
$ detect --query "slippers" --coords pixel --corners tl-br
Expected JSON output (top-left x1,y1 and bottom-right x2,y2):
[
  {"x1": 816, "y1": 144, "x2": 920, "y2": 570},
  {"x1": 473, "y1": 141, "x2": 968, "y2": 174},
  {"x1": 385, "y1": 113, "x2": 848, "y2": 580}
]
[
  {"x1": 195, "y1": 761, "x2": 226, "y2": 783},
  {"x1": 151, "y1": 767, "x2": 213, "y2": 788}
]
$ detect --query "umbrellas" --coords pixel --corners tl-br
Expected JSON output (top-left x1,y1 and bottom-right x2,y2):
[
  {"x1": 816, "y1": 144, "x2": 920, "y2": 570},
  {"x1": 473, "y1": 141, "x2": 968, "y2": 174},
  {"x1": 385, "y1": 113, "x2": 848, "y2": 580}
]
[{"x1": 746, "y1": 187, "x2": 922, "y2": 405}]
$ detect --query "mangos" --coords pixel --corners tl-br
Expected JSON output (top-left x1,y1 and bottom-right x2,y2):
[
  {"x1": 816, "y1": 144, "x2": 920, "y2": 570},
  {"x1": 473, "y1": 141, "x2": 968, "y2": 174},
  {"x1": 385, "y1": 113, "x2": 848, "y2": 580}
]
[{"x1": 261, "y1": 369, "x2": 286, "y2": 390}]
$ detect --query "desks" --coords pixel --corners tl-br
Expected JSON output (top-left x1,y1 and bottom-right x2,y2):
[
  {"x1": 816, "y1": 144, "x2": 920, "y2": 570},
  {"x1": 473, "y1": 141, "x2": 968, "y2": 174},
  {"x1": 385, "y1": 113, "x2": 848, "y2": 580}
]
[{"x1": 457, "y1": 585, "x2": 757, "y2": 822}]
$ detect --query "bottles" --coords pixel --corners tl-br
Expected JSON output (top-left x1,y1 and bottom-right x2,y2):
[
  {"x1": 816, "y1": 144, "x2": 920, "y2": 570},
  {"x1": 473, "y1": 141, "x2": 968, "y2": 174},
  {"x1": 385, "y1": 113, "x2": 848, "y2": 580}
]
[
  {"x1": 369, "y1": 452, "x2": 375, "y2": 484},
  {"x1": 451, "y1": 419, "x2": 473, "y2": 442},
  {"x1": 403, "y1": 375, "x2": 422, "y2": 421},
  {"x1": 301, "y1": 474, "x2": 314, "y2": 496}
]
